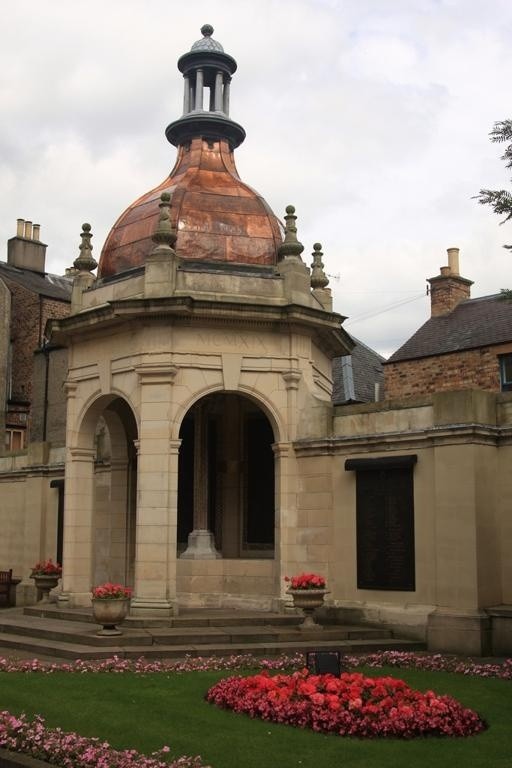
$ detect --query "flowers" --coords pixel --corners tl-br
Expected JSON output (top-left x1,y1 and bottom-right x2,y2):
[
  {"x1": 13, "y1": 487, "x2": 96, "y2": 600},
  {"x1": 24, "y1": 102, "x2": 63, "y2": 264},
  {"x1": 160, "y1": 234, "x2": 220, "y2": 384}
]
[
  {"x1": 281, "y1": 570, "x2": 328, "y2": 588},
  {"x1": 27, "y1": 557, "x2": 62, "y2": 574},
  {"x1": 90, "y1": 580, "x2": 131, "y2": 597}
]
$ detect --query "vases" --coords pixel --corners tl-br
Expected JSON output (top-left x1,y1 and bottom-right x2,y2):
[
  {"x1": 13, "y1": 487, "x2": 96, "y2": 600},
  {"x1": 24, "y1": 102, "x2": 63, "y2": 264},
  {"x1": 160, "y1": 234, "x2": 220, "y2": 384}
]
[
  {"x1": 27, "y1": 575, "x2": 60, "y2": 602},
  {"x1": 284, "y1": 590, "x2": 332, "y2": 629},
  {"x1": 89, "y1": 598, "x2": 131, "y2": 635}
]
